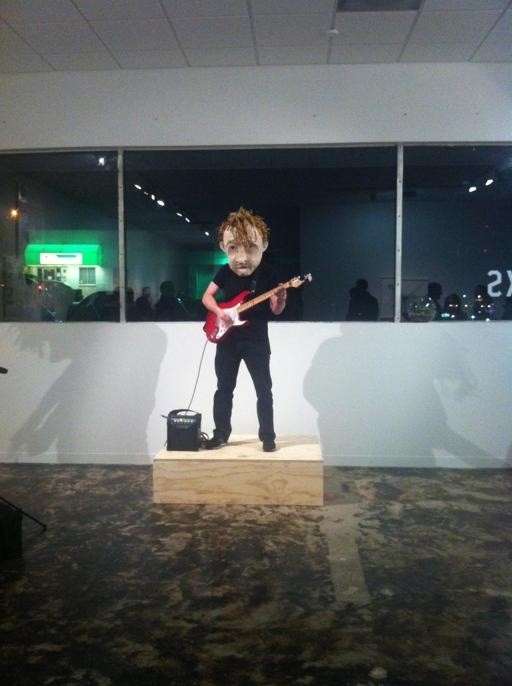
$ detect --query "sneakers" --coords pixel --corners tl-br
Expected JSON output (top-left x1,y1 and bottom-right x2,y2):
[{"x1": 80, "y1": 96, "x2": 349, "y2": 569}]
[
  {"x1": 203, "y1": 435, "x2": 227, "y2": 450},
  {"x1": 261, "y1": 436, "x2": 276, "y2": 453}
]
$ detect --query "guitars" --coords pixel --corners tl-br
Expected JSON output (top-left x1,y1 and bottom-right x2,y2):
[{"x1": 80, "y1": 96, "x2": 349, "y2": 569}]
[{"x1": 204, "y1": 274, "x2": 312, "y2": 342}]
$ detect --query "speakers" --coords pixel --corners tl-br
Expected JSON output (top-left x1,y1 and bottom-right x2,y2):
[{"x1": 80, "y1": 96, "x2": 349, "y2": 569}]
[{"x1": 166, "y1": 409, "x2": 202, "y2": 452}]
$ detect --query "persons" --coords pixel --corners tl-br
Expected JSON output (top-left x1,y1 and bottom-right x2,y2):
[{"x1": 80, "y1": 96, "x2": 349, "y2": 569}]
[{"x1": 201, "y1": 206, "x2": 288, "y2": 452}]
